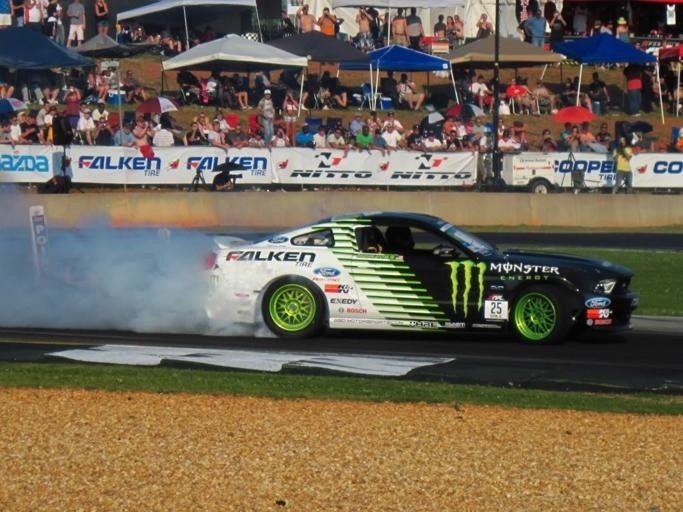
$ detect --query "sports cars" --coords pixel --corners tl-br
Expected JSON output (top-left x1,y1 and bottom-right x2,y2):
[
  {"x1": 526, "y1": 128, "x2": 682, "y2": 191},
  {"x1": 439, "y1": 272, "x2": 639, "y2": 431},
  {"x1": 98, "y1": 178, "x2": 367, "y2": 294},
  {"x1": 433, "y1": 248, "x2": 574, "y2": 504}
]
[{"x1": 198, "y1": 210, "x2": 639, "y2": 345}]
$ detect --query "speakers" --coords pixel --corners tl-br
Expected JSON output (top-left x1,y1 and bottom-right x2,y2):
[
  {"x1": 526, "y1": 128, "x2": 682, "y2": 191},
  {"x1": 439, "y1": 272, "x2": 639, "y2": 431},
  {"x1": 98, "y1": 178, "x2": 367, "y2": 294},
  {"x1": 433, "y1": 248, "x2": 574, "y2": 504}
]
[{"x1": 52, "y1": 117, "x2": 74, "y2": 145}]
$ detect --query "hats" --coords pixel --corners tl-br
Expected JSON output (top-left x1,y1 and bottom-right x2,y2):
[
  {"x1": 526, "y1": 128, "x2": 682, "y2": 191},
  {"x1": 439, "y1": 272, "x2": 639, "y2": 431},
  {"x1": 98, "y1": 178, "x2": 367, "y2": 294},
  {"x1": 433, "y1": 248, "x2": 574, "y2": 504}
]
[{"x1": 29, "y1": 0, "x2": 627, "y2": 146}]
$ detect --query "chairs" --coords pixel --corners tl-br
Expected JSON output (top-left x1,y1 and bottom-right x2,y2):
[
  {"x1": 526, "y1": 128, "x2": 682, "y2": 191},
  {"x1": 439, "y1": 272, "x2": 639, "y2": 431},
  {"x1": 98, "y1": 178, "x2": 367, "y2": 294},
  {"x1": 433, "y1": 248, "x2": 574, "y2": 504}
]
[
  {"x1": 509, "y1": 86, "x2": 540, "y2": 116},
  {"x1": 326, "y1": 117, "x2": 342, "y2": 134},
  {"x1": 305, "y1": 117, "x2": 322, "y2": 133},
  {"x1": 352, "y1": 83, "x2": 392, "y2": 111},
  {"x1": 177, "y1": 74, "x2": 253, "y2": 106}
]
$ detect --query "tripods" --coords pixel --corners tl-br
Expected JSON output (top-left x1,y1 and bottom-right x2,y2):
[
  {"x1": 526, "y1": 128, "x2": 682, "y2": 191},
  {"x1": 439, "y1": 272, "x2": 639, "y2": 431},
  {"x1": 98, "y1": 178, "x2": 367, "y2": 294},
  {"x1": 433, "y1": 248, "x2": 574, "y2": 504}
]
[
  {"x1": 42, "y1": 146, "x2": 84, "y2": 193},
  {"x1": 188, "y1": 172, "x2": 210, "y2": 192},
  {"x1": 560, "y1": 152, "x2": 587, "y2": 194}
]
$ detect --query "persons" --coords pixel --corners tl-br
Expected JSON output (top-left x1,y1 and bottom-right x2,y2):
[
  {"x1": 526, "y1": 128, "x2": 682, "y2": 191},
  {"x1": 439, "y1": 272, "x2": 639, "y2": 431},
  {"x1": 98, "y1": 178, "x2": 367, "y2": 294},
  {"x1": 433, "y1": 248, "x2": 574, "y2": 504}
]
[{"x1": 0, "y1": 2, "x2": 683, "y2": 193}]
